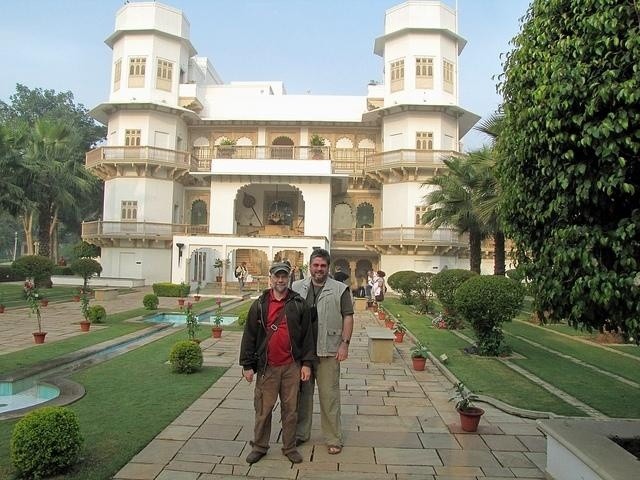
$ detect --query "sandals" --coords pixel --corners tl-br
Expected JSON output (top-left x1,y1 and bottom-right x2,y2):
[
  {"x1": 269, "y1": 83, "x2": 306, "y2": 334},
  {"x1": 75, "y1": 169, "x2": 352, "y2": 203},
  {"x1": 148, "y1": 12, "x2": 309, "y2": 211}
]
[
  {"x1": 327, "y1": 444, "x2": 343, "y2": 455},
  {"x1": 296, "y1": 437, "x2": 305, "y2": 447}
]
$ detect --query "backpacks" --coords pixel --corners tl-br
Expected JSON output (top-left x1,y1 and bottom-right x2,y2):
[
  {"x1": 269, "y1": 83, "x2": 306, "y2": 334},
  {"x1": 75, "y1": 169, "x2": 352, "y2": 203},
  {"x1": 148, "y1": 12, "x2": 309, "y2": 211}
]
[{"x1": 235, "y1": 265, "x2": 243, "y2": 278}]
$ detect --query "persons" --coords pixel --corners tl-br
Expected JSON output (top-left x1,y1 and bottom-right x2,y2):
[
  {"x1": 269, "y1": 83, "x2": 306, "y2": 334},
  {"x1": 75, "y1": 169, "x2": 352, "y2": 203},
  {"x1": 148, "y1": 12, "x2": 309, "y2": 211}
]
[
  {"x1": 58, "y1": 257, "x2": 67, "y2": 266},
  {"x1": 282, "y1": 257, "x2": 385, "y2": 305},
  {"x1": 236, "y1": 262, "x2": 249, "y2": 297},
  {"x1": 288, "y1": 249, "x2": 355, "y2": 454},
  {"x1": 239, "y1": 262, "x2": 314, "y2": 463}
]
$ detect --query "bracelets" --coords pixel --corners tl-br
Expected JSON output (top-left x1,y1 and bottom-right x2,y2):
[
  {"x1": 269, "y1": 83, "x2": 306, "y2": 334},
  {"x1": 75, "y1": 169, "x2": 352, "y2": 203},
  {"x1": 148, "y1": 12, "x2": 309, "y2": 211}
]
[{"x1": 341, "y1": 338, "x2": 350, "y2": 346}]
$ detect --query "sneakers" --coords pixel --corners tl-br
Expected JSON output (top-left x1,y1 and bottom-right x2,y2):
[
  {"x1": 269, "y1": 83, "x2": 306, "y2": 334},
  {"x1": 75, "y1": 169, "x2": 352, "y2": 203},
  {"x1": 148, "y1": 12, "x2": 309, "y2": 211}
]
[
  {"x1": 285, "y1": 450, "x2": 304, "y2": 464},
  {"x1": 246, "y1": 449, "x2": 267, "y2": 464}
]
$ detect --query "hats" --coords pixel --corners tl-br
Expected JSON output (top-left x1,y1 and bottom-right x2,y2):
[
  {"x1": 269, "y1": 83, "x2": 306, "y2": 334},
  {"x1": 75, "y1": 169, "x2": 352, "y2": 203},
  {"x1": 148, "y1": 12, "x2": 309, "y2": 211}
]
[{"x1": 267, "y1": 262, "x2": 292, "y2": 276}]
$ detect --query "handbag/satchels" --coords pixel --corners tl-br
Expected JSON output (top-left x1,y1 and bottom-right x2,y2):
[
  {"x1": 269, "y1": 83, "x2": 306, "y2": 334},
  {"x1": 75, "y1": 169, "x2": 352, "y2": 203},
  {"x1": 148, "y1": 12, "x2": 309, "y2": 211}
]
[
  {"x1": 241, "y1": 353, "x2": 260, "y2": 376},
  {"x1": 376, "y1": 292, "x2": 385, "y2": 301}
]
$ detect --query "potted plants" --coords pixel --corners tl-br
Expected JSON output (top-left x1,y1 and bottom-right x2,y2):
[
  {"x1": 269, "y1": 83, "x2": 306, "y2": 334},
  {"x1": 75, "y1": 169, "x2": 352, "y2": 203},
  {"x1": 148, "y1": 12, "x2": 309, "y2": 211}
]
[
  {"x1": 185, "y1": 309, "x2": 201, "y2": 345},
  {"x1": 194, "y1": 286, "x2": 201, "y2": 301},
  {"x1": 177, "y1": 281, "x2": 185, "y2": 305},
  {"x1": 378, "y1": 306, "x2": 406, "y2": 342},
  {"x1": 24, "y1": 290, "x2": 47, "y2": 343},
  {"x1": 447, "y1": 381, "x2": 485, "y2": 433},
  {"x1": 212, "y1": 308, "x2": 222, "y2": 338},
  {"x1": 309, "y1": 134, "x2": 326, "y2": 160},
  {"x1": 218, "y1": 139, "x2": 239, "y2": 158},
  {"x1": 212, "y1": 258, "x2": 222, "y2": 282},
  {"x1": 409, "y1": 340, "x2": 432, "y2": 371},
  {"x1": 79, "y1": 295, "x2": 91, "y2": 331},
  {"x1": 0, "y1": 304, "x2": 5, "y2": 313}
]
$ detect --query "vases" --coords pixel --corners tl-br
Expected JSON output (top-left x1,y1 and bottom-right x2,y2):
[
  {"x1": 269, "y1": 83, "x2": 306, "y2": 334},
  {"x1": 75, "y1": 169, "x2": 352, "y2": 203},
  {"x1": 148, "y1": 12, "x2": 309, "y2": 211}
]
[
  {"x1": 42, "y1": 300, "x2": 47, "y2": 306},
  {"x1": 75, "y1": 296, "x2": 80, "y2": 302}
]
